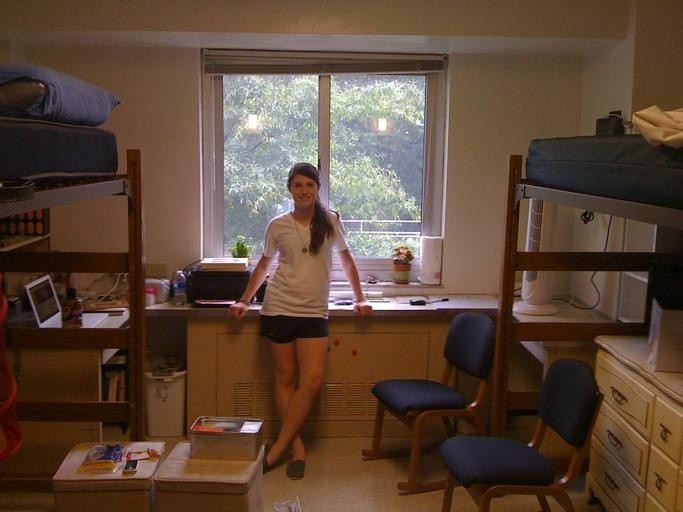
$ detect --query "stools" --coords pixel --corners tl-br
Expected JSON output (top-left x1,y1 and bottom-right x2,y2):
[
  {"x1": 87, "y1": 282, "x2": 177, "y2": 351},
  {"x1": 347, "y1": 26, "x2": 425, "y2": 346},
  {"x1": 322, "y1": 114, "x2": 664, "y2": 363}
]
[
  {"x1": 156, "y1": 438, "x2": 267, "y2": 512},
  {"x1": 52, "y1": 440, "x2": 164, "y2": 512}
]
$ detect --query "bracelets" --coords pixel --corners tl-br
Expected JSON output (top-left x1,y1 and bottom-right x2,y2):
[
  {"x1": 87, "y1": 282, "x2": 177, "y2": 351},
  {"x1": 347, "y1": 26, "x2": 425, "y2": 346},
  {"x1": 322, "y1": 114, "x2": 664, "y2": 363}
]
[{"x1": 236, "y1": 298, "x2": 250, "y2": 306}]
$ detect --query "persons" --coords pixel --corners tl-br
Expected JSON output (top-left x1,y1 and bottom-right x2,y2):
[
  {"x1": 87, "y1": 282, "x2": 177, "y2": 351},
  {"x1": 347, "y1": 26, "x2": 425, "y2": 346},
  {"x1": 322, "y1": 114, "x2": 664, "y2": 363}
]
[{"x1": 224, "y1": 160, "x2": 375, "y2": 482}]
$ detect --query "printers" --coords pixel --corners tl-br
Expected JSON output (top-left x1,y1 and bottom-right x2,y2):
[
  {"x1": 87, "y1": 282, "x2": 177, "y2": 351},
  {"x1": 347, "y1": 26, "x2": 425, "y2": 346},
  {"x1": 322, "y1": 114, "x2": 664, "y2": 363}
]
[{"x1": 182, "y1": 256, "x2": 257, "y2": 308}]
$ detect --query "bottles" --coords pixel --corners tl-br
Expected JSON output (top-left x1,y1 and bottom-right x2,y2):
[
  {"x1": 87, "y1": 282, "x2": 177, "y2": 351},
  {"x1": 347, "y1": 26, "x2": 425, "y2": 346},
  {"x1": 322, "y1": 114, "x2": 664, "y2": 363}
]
[
  {"x1": 60, "y1": 288, "x2": 82, "y2": 328},
  {"x1": 172, "y1": 270, "x2": 187, "y2": 306},
  {"x1": 145, "y1": 287, "x2": 155, "y2": 306}
]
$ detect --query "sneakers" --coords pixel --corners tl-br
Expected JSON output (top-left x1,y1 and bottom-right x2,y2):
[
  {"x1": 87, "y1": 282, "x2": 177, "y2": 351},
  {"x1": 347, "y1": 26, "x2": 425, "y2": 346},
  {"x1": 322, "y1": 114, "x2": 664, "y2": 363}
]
[{"x1": 262, "y1": 443, "x2": 306, "y2": 481}]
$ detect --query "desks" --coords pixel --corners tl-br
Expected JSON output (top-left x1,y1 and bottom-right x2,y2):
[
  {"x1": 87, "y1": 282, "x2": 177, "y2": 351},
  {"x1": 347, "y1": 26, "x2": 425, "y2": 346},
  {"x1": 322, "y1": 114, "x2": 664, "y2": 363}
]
[
  {"x1": 148, "y1": 287, "x2": 513, "y2": 443},
  {"x1": 507, "y1": 294, "x2": 616, "y2": 461},
  {"x1": 0, "y1": 290, "x2": 136, "y2": 493}
]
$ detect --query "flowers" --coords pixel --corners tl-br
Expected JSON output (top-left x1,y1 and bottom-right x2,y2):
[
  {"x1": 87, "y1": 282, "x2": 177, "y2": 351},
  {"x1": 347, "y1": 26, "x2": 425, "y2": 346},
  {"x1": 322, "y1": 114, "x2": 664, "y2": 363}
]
[
  {"x1": 389, "y1": 245, "x2": 412, "y2": 263},
  {"x1": 227, "y1": 233, "x2": 255, "y2": 257}
]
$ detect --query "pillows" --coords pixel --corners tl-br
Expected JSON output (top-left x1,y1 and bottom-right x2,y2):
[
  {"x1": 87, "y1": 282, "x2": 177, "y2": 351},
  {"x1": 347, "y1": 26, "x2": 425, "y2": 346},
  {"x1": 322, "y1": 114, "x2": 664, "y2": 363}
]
[{"x1": 0, "y1": 60, "x2": 121, "y2": 127}]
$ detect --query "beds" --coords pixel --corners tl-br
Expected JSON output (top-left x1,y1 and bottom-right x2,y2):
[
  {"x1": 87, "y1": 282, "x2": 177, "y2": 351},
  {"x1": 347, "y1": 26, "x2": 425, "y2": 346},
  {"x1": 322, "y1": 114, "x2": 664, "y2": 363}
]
[
  {"x1": 492, "y1": 118, "x2": 682, "y2": 437},
  {"x1": 0, "y1": 108, "x2": 149, "y2": 512}
]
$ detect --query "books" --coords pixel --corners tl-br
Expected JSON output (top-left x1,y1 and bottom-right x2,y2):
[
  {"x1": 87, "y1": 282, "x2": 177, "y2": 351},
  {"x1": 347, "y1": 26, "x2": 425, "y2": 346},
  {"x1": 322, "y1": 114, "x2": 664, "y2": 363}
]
[{"x1": 75, "y1": 444, "x2": 122, "y2": 475}]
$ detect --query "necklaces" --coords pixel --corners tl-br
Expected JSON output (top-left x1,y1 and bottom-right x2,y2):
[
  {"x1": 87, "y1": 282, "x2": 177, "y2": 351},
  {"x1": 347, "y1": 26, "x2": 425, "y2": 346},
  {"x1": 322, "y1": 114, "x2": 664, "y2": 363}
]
[{"x1": 290, "y1": 209, "x2": 323, "y2": 254}]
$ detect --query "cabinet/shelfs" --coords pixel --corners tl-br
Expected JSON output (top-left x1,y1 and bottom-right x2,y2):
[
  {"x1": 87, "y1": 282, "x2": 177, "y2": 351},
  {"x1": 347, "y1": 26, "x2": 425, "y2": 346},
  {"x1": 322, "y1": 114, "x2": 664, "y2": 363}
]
[
  {"x1": 0, "y1": 206, "x2": 56, "y2": 311},
  {"x1": 581, "y1": 329, "x2": 682, "y2": 512}
]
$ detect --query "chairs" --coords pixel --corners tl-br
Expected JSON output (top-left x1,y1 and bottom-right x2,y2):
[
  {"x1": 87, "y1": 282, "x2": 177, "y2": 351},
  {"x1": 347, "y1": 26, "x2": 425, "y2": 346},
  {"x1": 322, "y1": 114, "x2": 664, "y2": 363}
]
[
  {"x1": 433, "y1": 358, "x2": 604, "y2": 512},
  {"x1": 362, "y1": 313, "x2": 503, "y2": 492}
]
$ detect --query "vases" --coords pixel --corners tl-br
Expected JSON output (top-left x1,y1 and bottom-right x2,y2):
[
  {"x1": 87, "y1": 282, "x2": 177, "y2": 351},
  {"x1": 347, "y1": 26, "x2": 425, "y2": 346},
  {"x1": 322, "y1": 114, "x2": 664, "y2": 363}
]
[{"x1": 393, "y1": 262, "x2": 411, "y2": 284}]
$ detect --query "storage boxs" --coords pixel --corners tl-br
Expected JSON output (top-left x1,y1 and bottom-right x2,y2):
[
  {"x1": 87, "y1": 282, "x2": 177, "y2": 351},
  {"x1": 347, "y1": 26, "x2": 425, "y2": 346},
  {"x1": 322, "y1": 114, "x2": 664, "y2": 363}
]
[
  {"x1": 188, "y1": 416, "x2": 266, "y2": 461},
  {"x1": 184, "y1": 264, "x2": 257, "y2": 304}
]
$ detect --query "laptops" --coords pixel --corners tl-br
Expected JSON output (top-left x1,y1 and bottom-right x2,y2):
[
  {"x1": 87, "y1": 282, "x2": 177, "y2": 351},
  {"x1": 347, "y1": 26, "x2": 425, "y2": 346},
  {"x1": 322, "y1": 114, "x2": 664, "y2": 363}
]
[{"x1": 24, "y1": 275, "x2": 109, "y2": 328}]
